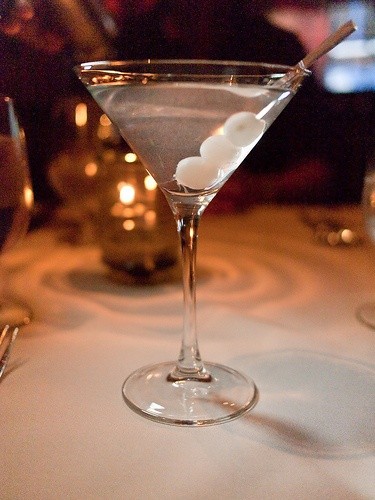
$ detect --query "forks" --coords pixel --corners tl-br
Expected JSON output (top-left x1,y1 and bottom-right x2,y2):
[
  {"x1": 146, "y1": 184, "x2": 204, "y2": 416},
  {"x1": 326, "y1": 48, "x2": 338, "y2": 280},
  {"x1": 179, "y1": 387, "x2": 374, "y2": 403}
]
[{"x1": 0, "y1": 324, "x2": 19, "y2": 379}]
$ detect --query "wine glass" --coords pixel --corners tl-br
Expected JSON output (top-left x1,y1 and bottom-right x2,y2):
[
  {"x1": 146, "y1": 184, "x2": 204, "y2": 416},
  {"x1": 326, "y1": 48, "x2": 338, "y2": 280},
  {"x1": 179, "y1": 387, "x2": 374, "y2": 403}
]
[
  {"x1": 358, "y1": 173, "x2": 375, "y2": 328},
  {"x1": 0, "y1": 96, "x2": 33, "y2": 326},
  {"x1": 76, "y1": 60, "x2": 312, "y2": 428}
]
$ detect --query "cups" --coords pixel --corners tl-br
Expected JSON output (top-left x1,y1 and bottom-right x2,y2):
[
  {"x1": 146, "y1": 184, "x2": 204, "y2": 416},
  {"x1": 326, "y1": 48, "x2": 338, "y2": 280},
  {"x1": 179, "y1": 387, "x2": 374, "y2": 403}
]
[{"x1": 97, "y1": 113, "x2": 181, "y2": 283}]
